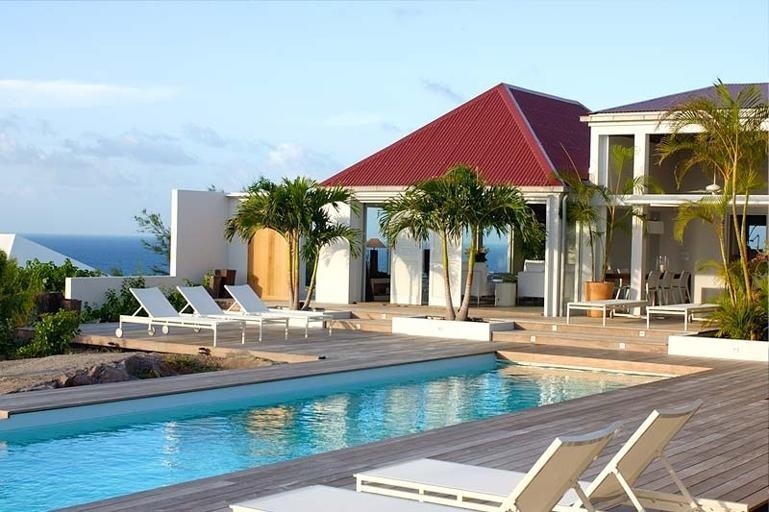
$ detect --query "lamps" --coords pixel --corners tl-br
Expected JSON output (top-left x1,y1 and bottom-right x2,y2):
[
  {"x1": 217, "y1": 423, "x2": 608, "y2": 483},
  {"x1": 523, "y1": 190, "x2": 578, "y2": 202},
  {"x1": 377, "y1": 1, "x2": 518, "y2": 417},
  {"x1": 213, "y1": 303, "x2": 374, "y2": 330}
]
[{"x1": 365, "y1": 238, "x2": 387, "y2": 271}]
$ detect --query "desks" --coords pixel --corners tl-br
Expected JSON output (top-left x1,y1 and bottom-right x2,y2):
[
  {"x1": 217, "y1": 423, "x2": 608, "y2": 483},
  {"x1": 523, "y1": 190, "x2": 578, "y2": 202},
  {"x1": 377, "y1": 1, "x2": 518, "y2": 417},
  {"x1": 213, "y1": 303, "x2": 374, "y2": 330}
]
[{"x1": 566, "y1": 299, "x2": 723, "y2": 330}]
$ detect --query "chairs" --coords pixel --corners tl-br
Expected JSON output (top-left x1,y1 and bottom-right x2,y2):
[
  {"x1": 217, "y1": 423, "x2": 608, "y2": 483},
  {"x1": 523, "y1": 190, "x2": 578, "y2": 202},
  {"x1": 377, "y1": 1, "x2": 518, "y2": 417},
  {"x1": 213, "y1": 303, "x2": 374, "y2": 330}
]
[
  {"x1": 115, "y1": 283, "x2": 333, "y2": 347},
  {"x1": 604, "y1": 267, "x2": 694, "y2": 313}
]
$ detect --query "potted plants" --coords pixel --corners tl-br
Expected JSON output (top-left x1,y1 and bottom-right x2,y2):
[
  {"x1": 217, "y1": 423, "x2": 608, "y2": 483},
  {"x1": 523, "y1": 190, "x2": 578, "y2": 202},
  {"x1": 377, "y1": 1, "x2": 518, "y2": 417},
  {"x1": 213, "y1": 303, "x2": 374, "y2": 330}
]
[{"x1": 543, "y1": 138, "x2": 667, "y2": 318}]
